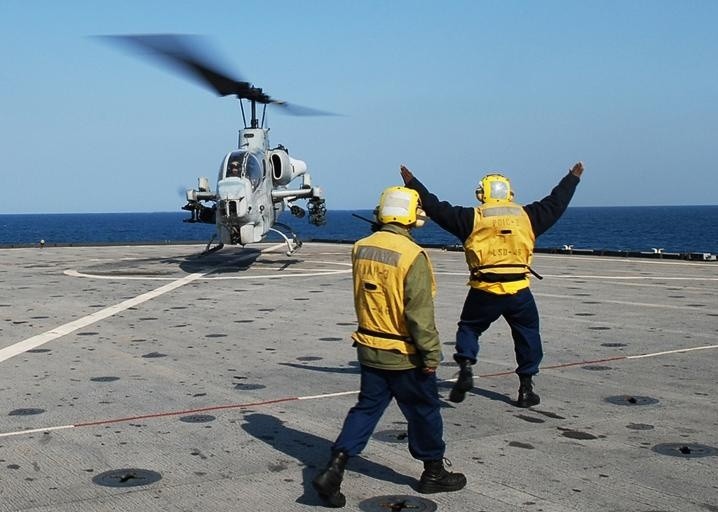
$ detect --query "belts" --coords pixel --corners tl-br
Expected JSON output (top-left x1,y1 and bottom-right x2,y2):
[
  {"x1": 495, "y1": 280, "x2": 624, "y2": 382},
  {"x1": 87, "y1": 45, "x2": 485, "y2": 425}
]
[{"x1": 471, "y1": 271, "x2": 533, "y2": 283}]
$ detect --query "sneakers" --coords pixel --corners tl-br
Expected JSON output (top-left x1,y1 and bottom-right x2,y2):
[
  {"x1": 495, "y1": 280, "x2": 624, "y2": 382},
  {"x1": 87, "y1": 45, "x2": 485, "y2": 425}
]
[
  {"x1": 517, "y1": 373, "x2": 540, "y2": 408},
  {"x1": 450, "y1": 359, "x2": 474, "y2": 403},
  {"x1": 419, "y1": 456, "x2": 467, "y2": 494},
  {"x1": 312, "y1": 449, "x2": 346, "y2": 508}
]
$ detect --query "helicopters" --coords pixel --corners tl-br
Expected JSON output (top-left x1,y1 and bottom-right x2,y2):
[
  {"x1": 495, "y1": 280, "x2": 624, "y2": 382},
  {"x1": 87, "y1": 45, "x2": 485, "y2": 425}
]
[{"x1": 83, "y1": 33, "x2": 345, "y2": 258}]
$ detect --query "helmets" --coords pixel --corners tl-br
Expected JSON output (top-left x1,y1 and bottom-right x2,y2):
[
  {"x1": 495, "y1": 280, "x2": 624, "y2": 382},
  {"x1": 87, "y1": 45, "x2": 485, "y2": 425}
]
[{"x1": 373, "y1": 186, "x2": 427, "y2": 228}]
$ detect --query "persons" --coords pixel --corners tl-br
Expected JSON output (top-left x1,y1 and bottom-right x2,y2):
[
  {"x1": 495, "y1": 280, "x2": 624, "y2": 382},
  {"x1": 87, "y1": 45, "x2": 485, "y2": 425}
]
[
  {"x1": 398, "y1": 159, "x2": 586, "y2": 409},
  {"x1": 311, "y1": 186, "x2": 467, "y2": 508}
]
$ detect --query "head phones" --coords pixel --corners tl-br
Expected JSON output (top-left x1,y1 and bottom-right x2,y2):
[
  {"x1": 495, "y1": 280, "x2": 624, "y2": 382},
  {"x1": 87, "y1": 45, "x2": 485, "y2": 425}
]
[{"x1": 374, "y1": 206, "x2": 427, "y2": 228}]
